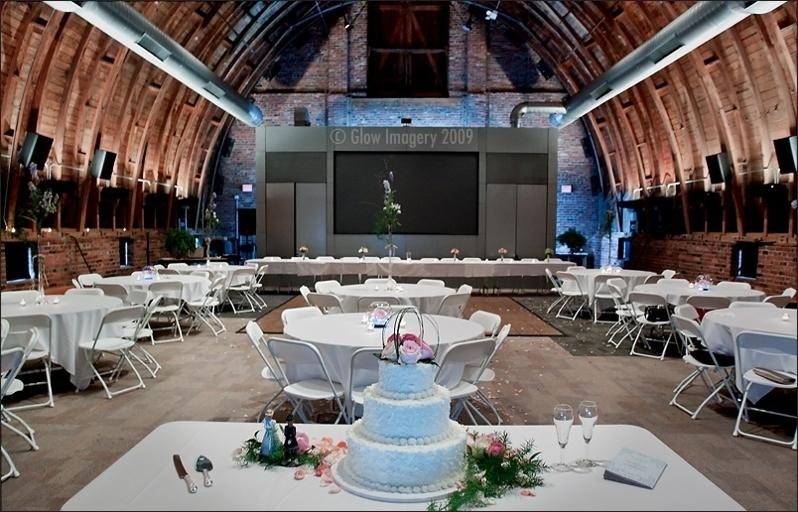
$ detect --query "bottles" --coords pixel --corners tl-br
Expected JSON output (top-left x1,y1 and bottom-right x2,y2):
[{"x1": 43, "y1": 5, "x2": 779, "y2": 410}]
[{"x1": 407, "y1": 251, "x2": 412, "y2": 262}]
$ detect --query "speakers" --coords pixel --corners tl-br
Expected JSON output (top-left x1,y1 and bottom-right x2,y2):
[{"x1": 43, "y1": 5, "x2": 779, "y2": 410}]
[
  {"x1": 773, "y1": 136, "x2": 797, "y2": 174},
  {"x1": 89, "y1": 149, "x2": 116, "y2": 181},
  {"x1": 17, "y1": 133, "x2": 53, "y2": 171},
  {"x1": 239, "y1": 208, "x2": 256, "y2": 235},
  {"x1": 705, "y1": 153, "x2": 731, "y2": 184}
]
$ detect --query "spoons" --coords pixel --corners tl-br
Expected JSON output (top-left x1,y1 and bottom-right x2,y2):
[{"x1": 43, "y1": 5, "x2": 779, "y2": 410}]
[{"x1": 193, "y1": 454, "x2": 216, "y2": 489}]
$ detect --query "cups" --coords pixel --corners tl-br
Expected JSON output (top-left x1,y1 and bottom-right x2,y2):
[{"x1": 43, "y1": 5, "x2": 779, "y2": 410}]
[
  {"x1": 577, "y1": 399, "x2": 601, "y2": 470},
  {"x1": 551, "y1": 402, "x2": 576, "y2": 474}
]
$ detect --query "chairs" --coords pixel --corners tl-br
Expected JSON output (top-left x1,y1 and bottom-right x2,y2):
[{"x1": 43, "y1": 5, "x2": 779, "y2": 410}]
[
  {"x1": 221, "y1": 267, "x2": 257, "y2": 315},
  {"x1": 64, "y1": 287, "x2": 105, "y2": 296},
  {"x1": 762, "y1": 292, "x2": 788, "y2": 309},
  {"x1": 166, "y1": 262, "x2": 189, "y2": 270},
  {"x1": 129, "y1": 290, "x2": 149, "y2": 308},
  {"x1": 417, "y1": 279, "x2": 446, "y2": 287},
  {"x1": 670, "y1": 314, "x2": 749, "y2": 423},
  {"x1": 347, "y1": 346, "x2": 383, "y2": 425},
  {"x1": 469, "y1": 309, "x2": 505, "y2": 338},
  {"x1": 644, "y1": 274, "x2": 665, "y2": 283},
  {"x1": 556, "y1": 270, "x2": 595, "y2": 322},
  {"x1": 1, "y1": 344, "x2": 25, "y2": 482},
  {"x1": 157, "y1": 267, "x2": 181, "y2": 276},
  {"x1": 0, "y1": 288, "x2": 45, "y2": 299},
  {"x1": 74, "y1": 304, "x2": 148, "y2": 399},
  {"x1": 0, "y1": 327, "x2": 40, "y2": 454},
  {"x1": 544, "y1": 268, "x2": 574, "y2": 317},
  {"x1": 91, "y1": 282, "x2": 128, "y2": 301},
  {"x1": 437, "y1": 293, "x2": 471, "y2": 319},
  {"x1": 153, "y1": 263, "x2": 166, "y2": 271},
  {"x1": 77, "y1": 271, "x2": 102, "y2": 287},
  {"x1": 435, "y1": 336, "x2": 498, "y2": 427},
  {"x1": 673, "y1": 303, "x2": 738, "y2": 394},
  {"x1": 450, "y1": 323, "x2": 513, "y2": 425},
  {"x1": 111, "y1": 294, "x2": 164, "y2": 380},
  {"x1": 70, "y1": 277, "x2": 82, "y2": 289},
  {"x1": 267, "y1": 336, "x2": 348, "y2": 424},
  {"x1": 364, "y1": 278, "x2": 397, "y2": 285},
  {"x1": 728, "y1": 300, "x2": 778, "y2": 308},
  {"x1": 314, "y1": 280, "x2": 342, "y2": 294},
  {"x1": 237, "y1": 264, "x2": 270, "y2": 310},
  {"x1": 356, "y1": 295, "x2": 402, "y2": 317},
  {"x1": 245, "y1": 319, "x2": 308, "y2": 422},
  {"x1": 281, "y1": 305, "x2": 326, "y2": 327},
  {"x1": 184, "y1": 275, "x2": 228, "y2": 338},
  {"x1": 590, "y1": 274, "x2": 625, "y2": 324},
  {"x1": 606, "y1": 278, "x2": 646, "y2": 351},
  {"x1": 299, "y1": 284, "x2": 311, "y2": 307},
  {"x1": 145, "y1": 280, "x2": 185, "y2": 346},
  {"x1": 306, "y1": 292, "x2": 345, "y2": 314},
  {"x1": 456, "y1": 283, "x2": 473, "y2": 295},
  {"x1": 733, "y1": 328, "x2": 798, "y2": 451},
  {"x1": 0, "y1": 313, "x2": 55, "y2": 412},
  {"x1": 781, "y1": 285, "x2": 798, "y2": 298},
  {"x1": 656, "y1": 278, "x2": 692, "y2": 286},
  {"x1": 717, "y1": 282, "x2": 753, "y2": 290},
  {"x1": 627, "y1": 291, "x2": 682, "y2": 362}
]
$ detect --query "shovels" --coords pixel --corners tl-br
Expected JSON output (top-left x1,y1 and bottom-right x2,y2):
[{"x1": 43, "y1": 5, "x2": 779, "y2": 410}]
[{"x1": 196, "y1": 454, "x2": 214, "y2": 487}]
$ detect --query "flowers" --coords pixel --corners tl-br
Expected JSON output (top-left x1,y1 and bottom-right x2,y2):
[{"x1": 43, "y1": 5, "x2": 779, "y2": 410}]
[
  {"x1": 544, "y1": 248, "x2": 555, "y2": 256},
  {"x1": 497, "y1": 247, "x2": 509, "y2": 255},
  {"x1": 425, "y1": 426, "x2": 555, "y2": 512},
  {"x1": 299, "y1": 247, "x2": 308, "y2": 253},
  {"x1": 358, "y1": 246, "x2": 369, "y2": 254},
  {"x1": 239, "y1": 424, "x2": 333, "y2": 469},
  {"x1": 450, "y1": 248, "x2": 460, "y2": 255},
  {"x1": 373, "y1": 171, "x2": 403, "y2": 242}
]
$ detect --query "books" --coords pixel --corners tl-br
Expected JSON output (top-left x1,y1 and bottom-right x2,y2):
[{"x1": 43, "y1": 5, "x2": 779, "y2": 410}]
[
  {"x1": 602, "y1": 446, "x2": 667, "y2": 489},
  {"x1": 753, "y1": 367, "x2": 796, "y2": 384}
]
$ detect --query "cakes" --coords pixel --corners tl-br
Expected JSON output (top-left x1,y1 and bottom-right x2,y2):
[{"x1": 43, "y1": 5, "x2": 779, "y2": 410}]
[{"x1": 342, "y1": 334, "x2": 467, "y2": 494}]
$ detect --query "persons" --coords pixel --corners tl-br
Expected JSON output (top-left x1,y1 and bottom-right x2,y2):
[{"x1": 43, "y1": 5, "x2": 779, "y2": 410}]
[
  {"x1": 259, "y1": 409, "x2": 282, "y2": 456},
  {"x1": 284, "y1": 415, "x2": 299, "y2": 455}
]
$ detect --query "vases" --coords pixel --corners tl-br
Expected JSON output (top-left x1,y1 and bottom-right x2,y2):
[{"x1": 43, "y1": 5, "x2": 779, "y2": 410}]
[
  {"x1": 361, "y1": 254, "x2": 366, "y2": 261},
  {"x1": 453, "y1": 255, "x2": 457, "y2": 262},
  {"x1": 547, "y1": 255, "x2": 551, "y2": 264},
  {"x1": 386, "y1": 225, "x2": 396, "y2": 290},
  {"x1": 500, "y1": 254, "x2": 504, "y2": 262},
  {"x1": 303, "y1": 252, "x2": 306, "y2": 260}
]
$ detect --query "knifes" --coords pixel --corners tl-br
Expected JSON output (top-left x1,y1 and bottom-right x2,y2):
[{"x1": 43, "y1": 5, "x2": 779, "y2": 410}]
[{"x1": 171, "y1": 452, "x2": 199, "y2": 495}]
